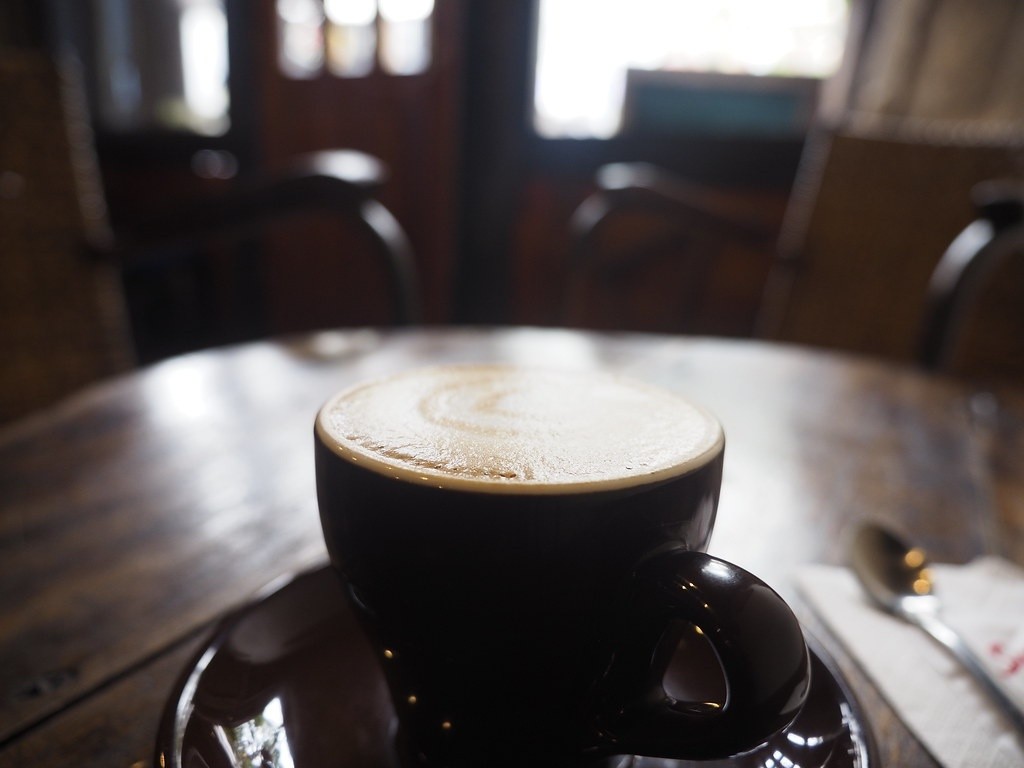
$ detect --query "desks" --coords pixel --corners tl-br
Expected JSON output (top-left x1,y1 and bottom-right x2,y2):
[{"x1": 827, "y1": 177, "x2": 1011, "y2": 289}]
[{"x1": 0, "y1": 327, "x2": 1024, "y2": 768}]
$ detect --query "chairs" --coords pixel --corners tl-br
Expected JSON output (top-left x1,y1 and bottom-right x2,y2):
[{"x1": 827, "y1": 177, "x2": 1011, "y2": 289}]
[
  {"x1": 549, "y1": 162, "x2": 774, "y2": 332},
  {"x1": 124, "y1": 150, "x2": 419, "y2": 370}
]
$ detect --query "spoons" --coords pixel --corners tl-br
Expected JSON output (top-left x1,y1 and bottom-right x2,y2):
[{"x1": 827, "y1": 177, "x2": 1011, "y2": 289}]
[{"x1": 838, "y1": 518, "x2": 1024, "y2": 742}]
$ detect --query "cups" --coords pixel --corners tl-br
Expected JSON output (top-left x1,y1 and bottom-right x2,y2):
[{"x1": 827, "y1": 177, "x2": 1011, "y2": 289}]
[{"x1": 313, "y1": 362, "x2": 814, "y2": 768}]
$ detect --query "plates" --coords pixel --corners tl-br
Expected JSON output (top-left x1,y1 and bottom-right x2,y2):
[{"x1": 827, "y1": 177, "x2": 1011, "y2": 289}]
[{"x1": 154, "y1": 546, "x2": 867, "y2": 767}]
